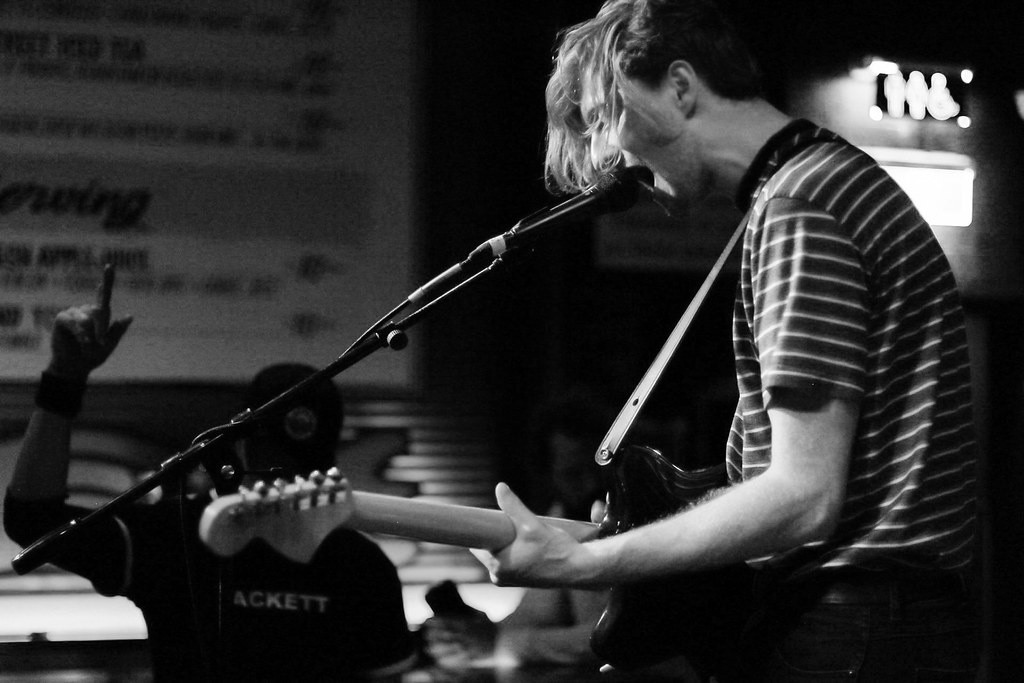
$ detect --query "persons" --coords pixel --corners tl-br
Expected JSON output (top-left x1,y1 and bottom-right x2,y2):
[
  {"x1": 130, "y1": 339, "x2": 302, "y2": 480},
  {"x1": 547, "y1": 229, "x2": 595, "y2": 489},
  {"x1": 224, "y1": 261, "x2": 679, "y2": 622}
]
[
  {"x1": 471, "y1": 0, "x2": 988, "y2": 682},
  {"x1": 0, "y1": 261, "x2": 410, "y2": 682}
]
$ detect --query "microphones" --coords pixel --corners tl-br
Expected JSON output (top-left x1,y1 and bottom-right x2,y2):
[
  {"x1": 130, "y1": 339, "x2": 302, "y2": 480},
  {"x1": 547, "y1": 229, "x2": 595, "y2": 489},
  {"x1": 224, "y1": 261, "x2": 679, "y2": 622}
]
[{"x1": 470, "y1": 171, "x2": 640, "y2": 263}]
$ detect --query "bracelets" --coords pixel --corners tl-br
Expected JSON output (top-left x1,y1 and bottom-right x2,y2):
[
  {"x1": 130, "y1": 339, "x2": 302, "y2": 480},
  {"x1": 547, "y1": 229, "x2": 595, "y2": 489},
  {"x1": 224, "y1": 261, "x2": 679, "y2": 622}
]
[{"x1": 36, "y1": 373, "x2": 86, "y2": 412}]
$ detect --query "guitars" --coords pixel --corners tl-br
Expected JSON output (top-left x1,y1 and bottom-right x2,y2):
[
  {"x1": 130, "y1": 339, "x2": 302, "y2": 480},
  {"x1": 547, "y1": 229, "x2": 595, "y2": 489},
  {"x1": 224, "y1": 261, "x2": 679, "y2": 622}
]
[{"x1": 197, "y1": 440, "x2": 729, "y2": 682}]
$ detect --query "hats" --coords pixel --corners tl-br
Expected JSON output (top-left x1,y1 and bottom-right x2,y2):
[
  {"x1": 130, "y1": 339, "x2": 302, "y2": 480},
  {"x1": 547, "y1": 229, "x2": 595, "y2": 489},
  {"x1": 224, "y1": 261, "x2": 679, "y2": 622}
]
[{"x1": 242, "y1": 359, "x2": 344, "y2": 478}]
